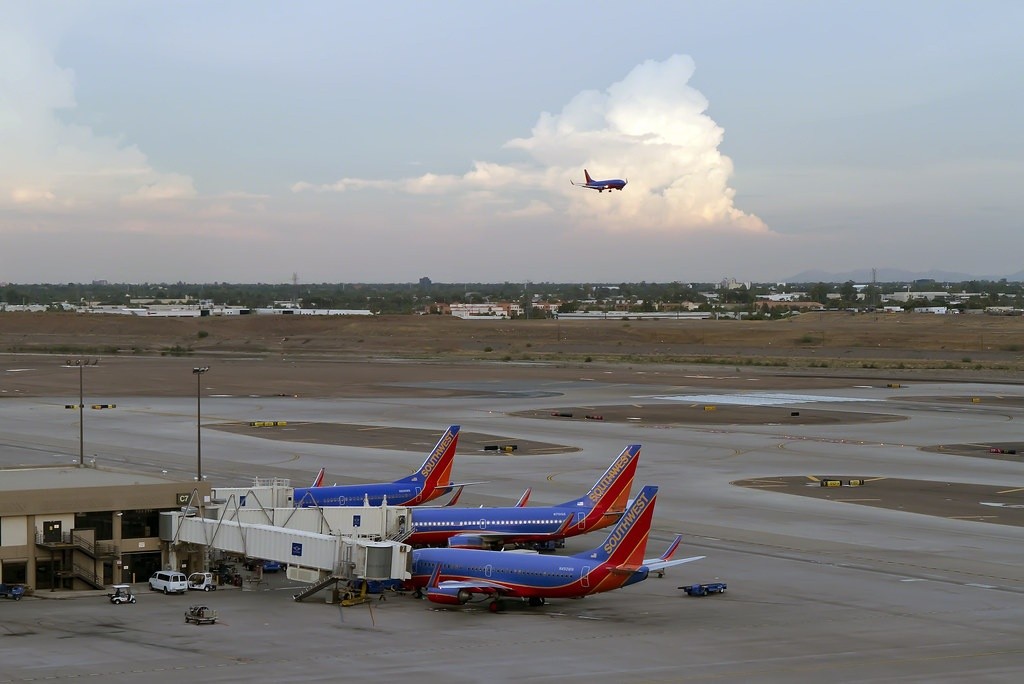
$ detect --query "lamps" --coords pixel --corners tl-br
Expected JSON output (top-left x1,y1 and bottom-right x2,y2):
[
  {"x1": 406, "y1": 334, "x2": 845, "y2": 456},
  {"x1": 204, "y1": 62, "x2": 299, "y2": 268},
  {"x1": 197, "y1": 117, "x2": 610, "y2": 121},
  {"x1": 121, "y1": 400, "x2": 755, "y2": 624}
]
[{"x1": 116, "y1": 512, "x2": 124, "y2": 516}]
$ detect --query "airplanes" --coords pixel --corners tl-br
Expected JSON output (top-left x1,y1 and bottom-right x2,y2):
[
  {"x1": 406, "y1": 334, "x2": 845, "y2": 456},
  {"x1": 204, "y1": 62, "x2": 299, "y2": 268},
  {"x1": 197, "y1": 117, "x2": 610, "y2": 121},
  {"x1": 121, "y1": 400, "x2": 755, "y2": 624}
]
[
  {"x1": 404, "y1": 444, "x2": 708, "y2": 611},
  {"x1": 290, "y1": 425, "x2": 490, "y2": 506},
  {"x1": 570, "y1": 170, "x2": 628, "y2": 192}
]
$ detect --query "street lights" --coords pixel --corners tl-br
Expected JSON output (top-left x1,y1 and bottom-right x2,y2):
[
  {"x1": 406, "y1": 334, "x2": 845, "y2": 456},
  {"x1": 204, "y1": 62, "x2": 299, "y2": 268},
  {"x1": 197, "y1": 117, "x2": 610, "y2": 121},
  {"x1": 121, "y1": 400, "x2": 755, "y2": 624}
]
[
  {"x1": 193, "y1": 366, "x2": 209, "y2": 481},
  {"x1": 66, "y1": 359, "x2": 101, "y2": 465}
]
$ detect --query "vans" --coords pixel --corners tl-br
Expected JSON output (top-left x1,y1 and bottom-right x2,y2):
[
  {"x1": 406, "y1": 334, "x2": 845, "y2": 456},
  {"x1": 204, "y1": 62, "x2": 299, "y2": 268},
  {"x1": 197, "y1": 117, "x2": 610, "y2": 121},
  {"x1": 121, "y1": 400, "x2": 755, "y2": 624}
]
[{"x1": 149, "y1": 571, "x2": 187, "y2": 595}]
